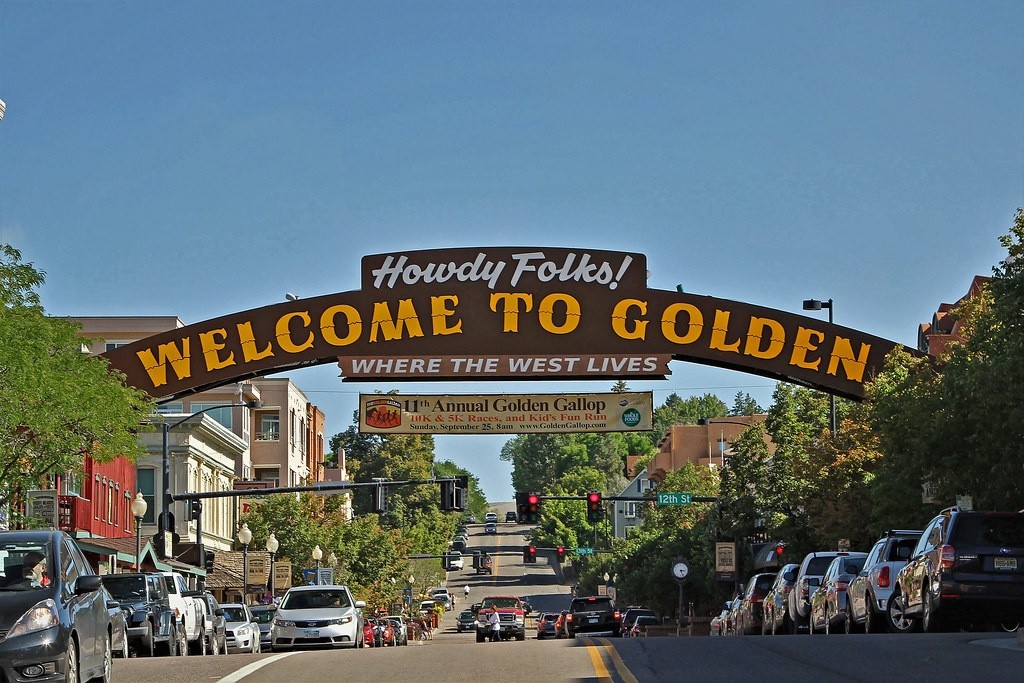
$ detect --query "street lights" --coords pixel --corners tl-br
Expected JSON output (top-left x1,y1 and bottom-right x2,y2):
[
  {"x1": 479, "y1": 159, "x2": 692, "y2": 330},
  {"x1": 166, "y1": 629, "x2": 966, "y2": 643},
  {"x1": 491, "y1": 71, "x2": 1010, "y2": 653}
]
[
  {"x1": 312, "y1": 544, "x2": 323, "y2": 585},
  {"x1": 266, "y1": 532, "x2": 279, "y2": 604},
  {"x1": 409, "y1": 574, "x2": 415, "y2": 624},
  {"x1": 238, "y1": 522, "x2": 252, "y2": 606},
  {"x1": 802, "y1": 298, "x2": 841, "y2": 551},
  {"x1": 129, "y1": 492, "x2": 148, "y2": 573},
  {"x1": 604, "y1": 572, "x2": 610, "y2": 595}
]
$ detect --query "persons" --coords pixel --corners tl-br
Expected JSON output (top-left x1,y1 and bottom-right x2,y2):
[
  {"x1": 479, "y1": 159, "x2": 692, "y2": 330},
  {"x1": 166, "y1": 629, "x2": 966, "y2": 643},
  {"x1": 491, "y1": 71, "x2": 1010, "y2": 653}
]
[
  {"x1": 464, "y1": 584, "x2": 470, "y2": 599},
  {"x1": 449, "y1": 592, "x2": 455, "y2": 611},
  {"x1": 489, "y1": 605, "x2": 502, "y2": 642},
  {"x1": 23, "y1": 552, "x2": 52, "y2": 587},
  {"x1": 223, "y1": 610, "x2": 232, "y2": 620},
  {"x1": 419, "y1": 617, "x2": 433, "y2": 640},
  {"x1": 333, "y1": 597, "x2": 341, "y2": 606}
]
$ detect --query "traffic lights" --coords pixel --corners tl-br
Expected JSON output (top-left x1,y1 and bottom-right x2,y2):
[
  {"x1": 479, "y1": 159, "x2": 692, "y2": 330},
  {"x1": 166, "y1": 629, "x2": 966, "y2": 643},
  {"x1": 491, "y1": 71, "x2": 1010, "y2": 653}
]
[
  {"x1": 523, "y1": 546, "x2": 536, "y2": 563},
  {"x1": 527, "y1": 494, "x2": 541, "y2": 523},
  {"x1": 557, "y1": 546, "x2": 564, "y2": 563},
  {"x1": 587, "y1": 492, "x2": 603, "y2": 522},
  {"x1": 205, "y1": 549, "x2": 215, "y2": 574},
  {"x1": 187, "y1": 492, "x2": 199, "y2": 521}
]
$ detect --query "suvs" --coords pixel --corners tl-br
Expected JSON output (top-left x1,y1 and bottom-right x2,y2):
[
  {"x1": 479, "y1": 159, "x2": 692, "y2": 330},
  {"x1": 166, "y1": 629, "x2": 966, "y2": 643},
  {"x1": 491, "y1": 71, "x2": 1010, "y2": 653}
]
[
  {"x1": 782, "y1": 550, "x2": 863, "y2": 635},
  {"x1": 560, "y1": 594, "x2": 621, "y2": 639},
  {"x1": 844, "y1": 530, "x2": 925, "y2": 634},
  {"x1": 94, "y1": 509, "x2": 667, "y2": 660},
  {"x1": 706, "y1": 571, "x2": 777, "y2": 637},
  {"x1": 472, "y1": 595, "x2": 533, "y2": 642},
  {"x1": 760, "y1": 563, "x2": 803, "y2": 635},
  {"x1": 883, "y1": 504, "x2": 1024, "y2": 633}
]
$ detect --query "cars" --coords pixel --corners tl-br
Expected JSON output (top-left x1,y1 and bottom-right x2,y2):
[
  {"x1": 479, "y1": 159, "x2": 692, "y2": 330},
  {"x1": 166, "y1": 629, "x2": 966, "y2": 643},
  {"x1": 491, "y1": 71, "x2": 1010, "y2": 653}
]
[
  {"x1": 0, "y1": 526, "x2": 118, "y2": 683},
  {"x1": 267, "y1": 584, "x2": 366, "y2": 654},
  {"x1": 808, "y1": 552, "x2": 870, "y2": 636}
]
